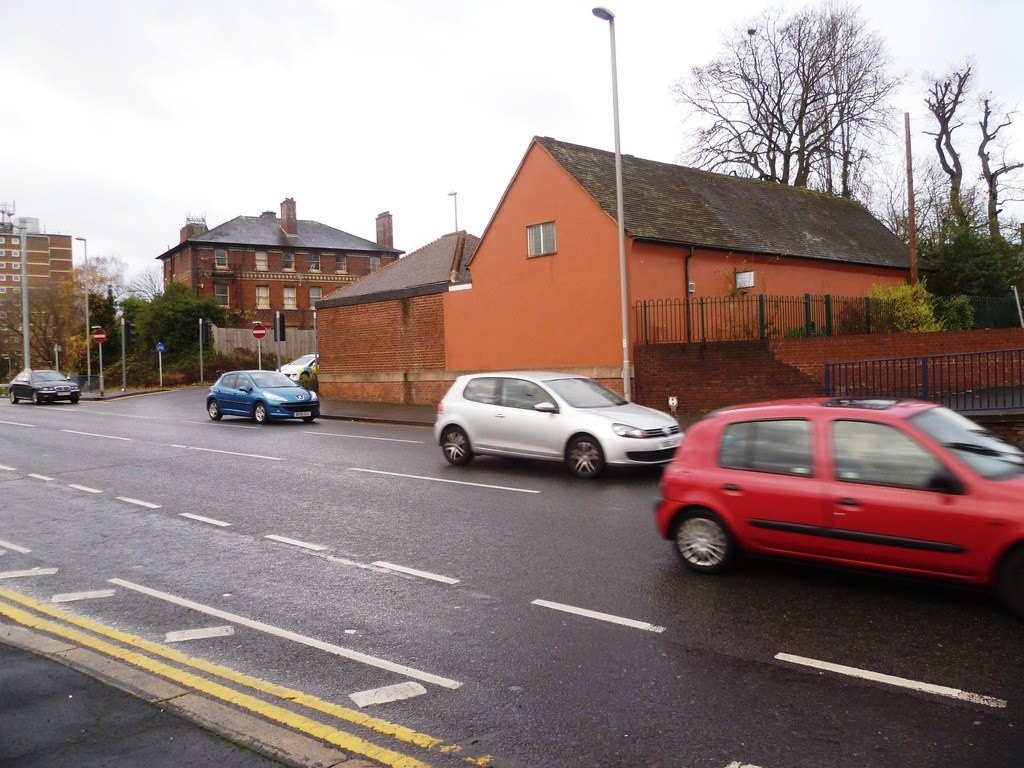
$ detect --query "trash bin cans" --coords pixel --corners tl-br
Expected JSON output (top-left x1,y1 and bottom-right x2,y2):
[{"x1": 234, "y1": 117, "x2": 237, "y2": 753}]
[{"x1": 67, "y1": 373, "x2": 80, "y2": 385}]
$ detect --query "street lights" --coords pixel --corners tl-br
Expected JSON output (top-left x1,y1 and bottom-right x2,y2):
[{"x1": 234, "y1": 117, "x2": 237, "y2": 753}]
[
  {"x1": 448, "y1": 192, "x2": 458, "y2": 232},
  {"x1": 92, "y1": 326, "x2": 104, "y2": 396},
  {"x1": 592, "y1": 8, "x2": 631, "y2": 404},
  {"x1": 4, "y1": 357, "x2": 11, "y2": 384},
  {"x1": 252, "y1": 321, "x2": 261, "y2": 370},
  {"x1": 75, "y1": 238, "x2": 92, "y2": 393}
]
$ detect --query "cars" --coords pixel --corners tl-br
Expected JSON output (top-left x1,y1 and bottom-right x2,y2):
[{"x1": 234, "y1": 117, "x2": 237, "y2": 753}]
[
  {"x1": 206, "y1": 370, "x2": 320, "y2": 425},
  {"x1": 8, "y1": 369, "x2": 82, "y2": 405},
  {"x1": 434, "y1": 371, "x2": 685, "y2": 480},
  {"x1": 652, "y1": 397, "x2": 1024, "y2": 607}
]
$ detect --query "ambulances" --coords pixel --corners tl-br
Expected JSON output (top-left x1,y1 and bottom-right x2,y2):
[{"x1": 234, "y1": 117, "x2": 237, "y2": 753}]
[{"x1": 276, "y1": 353, "x2": 319, "y2": 381}]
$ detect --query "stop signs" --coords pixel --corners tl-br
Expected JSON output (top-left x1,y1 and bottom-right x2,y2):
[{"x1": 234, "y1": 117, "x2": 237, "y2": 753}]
[
  {"x1": 252, "y1": 325, "x2": 266, "y2": 339},
  {"x1": 93, "y1": 329, "x2": 106, "y2": 343}
]
